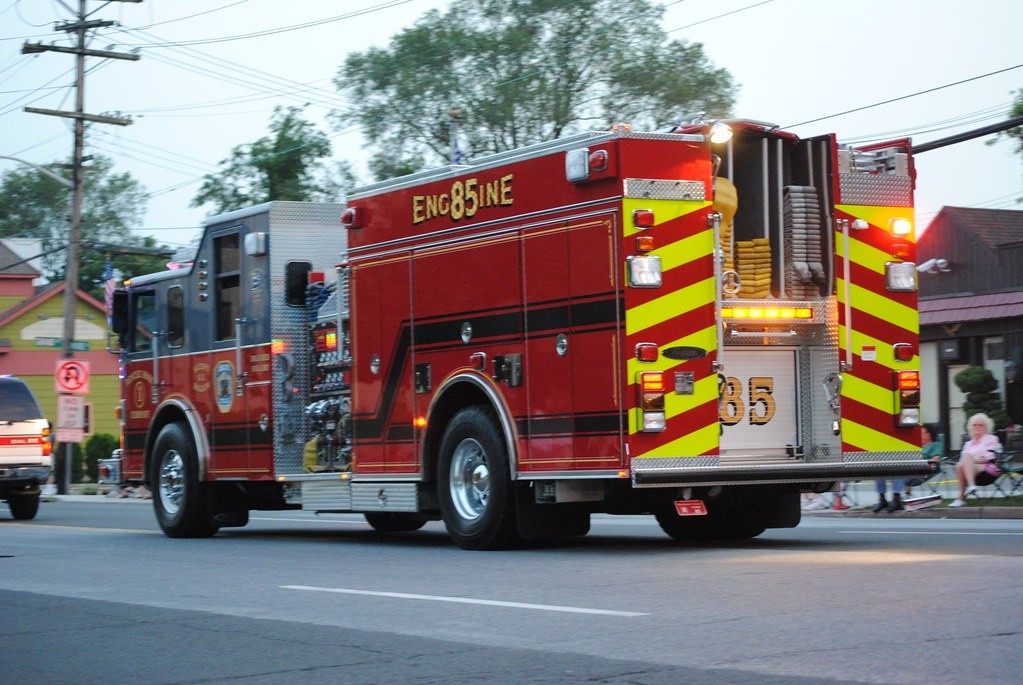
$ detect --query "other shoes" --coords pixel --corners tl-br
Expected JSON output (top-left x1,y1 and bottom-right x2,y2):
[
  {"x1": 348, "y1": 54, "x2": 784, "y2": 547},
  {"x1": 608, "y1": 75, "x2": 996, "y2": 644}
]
[
  {"x1": 948, "y1": 499, "x2": 967, "y2": 508},
  {"x1": 963, "y1": 485, "x2": 978, "y2": 497},
  {"x1": 873, "y1": 498, "x2": 887, "y2": 513},
  {"x1": 805, "y1": 497, "x2": 828, "y2": 511},
  {"x1": 887, "y1": 496, "x2": 903, "y2": 512}
]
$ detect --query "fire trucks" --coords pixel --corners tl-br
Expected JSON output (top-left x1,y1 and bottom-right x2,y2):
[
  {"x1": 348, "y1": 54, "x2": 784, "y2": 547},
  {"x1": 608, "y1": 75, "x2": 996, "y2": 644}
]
[{"x1": 105, "y1": 118, "x2": 942, "y2": 553}]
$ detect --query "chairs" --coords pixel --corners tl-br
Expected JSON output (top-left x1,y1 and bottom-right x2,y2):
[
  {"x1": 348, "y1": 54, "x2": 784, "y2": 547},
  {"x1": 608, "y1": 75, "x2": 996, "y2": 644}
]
[{"x1": 826, "y1": 449, "x2": 1023, "y2": 510}]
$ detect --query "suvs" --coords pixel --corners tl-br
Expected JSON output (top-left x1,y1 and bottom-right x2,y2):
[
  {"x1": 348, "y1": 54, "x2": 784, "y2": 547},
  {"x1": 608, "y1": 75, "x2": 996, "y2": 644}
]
[{"x1": 0, "y1": 372, "x2": 54, "y2": 521}]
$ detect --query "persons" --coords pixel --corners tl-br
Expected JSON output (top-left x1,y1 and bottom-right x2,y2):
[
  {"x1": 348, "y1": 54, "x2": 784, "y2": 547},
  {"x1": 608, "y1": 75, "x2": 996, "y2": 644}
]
[
  {"x1": 833, "y1": 483, "x2": 851, "y2": 511},
  {"x1": 801, "y1": 492, "x2": 826, "y2": 511},
  {"x1": 136, "y1": 306, "x2": 184, "y2": 343},
  {"x1": 948, "y1": 413, "x2": 1003, "y2": 507},
  {"x1": 872, "y1": 423, "x2": 944, "y2": 513}
]
